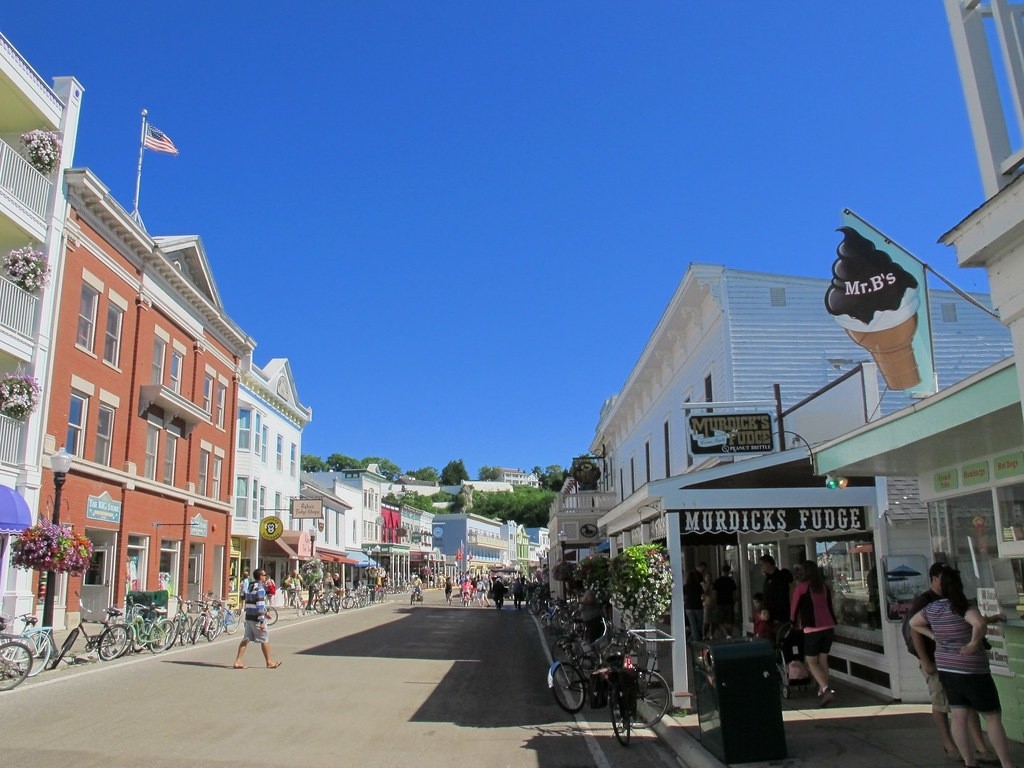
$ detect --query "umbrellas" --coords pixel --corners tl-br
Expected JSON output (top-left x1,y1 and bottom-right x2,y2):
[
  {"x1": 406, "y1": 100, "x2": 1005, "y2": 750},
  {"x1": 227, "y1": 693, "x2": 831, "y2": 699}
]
[{"x1": 887, "y1": 563, "x2": 922, "y2": 590}]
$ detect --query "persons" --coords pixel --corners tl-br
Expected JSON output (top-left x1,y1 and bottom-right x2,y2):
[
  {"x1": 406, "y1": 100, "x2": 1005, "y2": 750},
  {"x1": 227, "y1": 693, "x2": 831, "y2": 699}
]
[
  {"x1": 283, "y1": 570, "x2": 303, "y2": 608},
  {"x1": 751, "y1": 555, "x2": 838, "y2": 706},
  {"x1": 910, "y1": 565, "x2": 1021, "y2": 768},
  {"x1": 571, "y1": 581, "x2": 603, "y2": 670},
  {"x1": 445, "y1": 577, "x2": 452, "y2": 605},
  {"x1": 411, "y1": 575, "x2": 422, "y2": 594},
  {"x1": 685, "y1": 562, "x2": 738, "y2": 643},
  {"x1": 456, "y1": 576, "x2": 505, "y2": 611},
  {"x1": 314, "y1": 572, "x2": 341, "y2": 599},
  {"x1": 902, "y1": 561, "x2": 1008, "y2": 764},
  {"x1": 232, "y1": 569, "x2": 281, "y2": 669},
  {"x1": 511, "y1": 575, "x2": 528, "y2": 609}
]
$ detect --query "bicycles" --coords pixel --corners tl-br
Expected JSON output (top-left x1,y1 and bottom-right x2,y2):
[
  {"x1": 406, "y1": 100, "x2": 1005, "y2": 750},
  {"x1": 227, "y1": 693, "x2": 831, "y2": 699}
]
[
  {"x1": 526, "y1": 588, "x2": 671, "y2": 747},
  {"x1": 50, "y1": 590, "x2": 128, "y2": 669},
  {"x1": 0, "y1": 613, "x2": 54, "y2": 690},
  {"x1": 444, "y1": 587, "x2": 453, "y2": 606},
  {"x1": 290, "y1": 582, "x2": 387, "y2": 617},
  {"x1": 265, "y1": 605, "x2": 278, "y2": 625},
  {"x1": 103, "y1": 593, "x2": 244, "y2": 658},
  {"x1": 463, "y1": 592, "x2": 470, "y2": 606},
  {"x1": 458, "y1": 585, "x2": 476, "y2": 606}
]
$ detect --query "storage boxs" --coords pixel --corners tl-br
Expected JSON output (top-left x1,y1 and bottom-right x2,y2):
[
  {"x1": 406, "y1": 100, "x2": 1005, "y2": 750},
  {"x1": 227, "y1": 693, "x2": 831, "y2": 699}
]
[{"x1": 126, "y1": 590, "x2": 168, "y2": 649}]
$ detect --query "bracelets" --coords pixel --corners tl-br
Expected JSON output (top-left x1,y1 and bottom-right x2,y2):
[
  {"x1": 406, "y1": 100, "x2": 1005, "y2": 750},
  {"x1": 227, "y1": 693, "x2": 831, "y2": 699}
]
[{"x1": 932, "y1": 636, "x2": 934, "y2": 640}]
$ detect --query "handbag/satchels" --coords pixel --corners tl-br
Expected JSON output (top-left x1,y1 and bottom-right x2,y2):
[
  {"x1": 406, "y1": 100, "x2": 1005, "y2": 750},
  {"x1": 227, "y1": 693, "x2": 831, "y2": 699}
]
[{"x1": 267, "y1": 580, "x2": 275, "y2": 595}]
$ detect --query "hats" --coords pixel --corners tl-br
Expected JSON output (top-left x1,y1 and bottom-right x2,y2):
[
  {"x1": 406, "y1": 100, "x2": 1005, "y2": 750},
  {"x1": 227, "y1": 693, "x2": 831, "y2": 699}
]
[{"x1": 929, "y1": 562, "x2": 952, "y2": 576}]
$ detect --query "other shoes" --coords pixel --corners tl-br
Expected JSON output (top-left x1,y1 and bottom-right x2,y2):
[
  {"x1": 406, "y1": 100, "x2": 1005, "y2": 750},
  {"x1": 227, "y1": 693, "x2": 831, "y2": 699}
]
[
  {"x1": 945, "y1": 748, "x2": 963, "y2": 760},
  {"x1": 820, "y1": 687, "x2": 835, "y2": 706},
  {"x1": 974, "y1": 750, "x2": 999, "y2": 762}
]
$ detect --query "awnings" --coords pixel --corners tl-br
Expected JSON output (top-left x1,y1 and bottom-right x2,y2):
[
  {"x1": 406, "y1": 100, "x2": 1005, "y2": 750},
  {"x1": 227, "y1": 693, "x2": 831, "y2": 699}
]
[
  {"x1": 261, "y1": 529, "x2": 316, "y2": 560},
  {"x1": 320, "y1": 552, "x2": 359, "y2": 565},
  {"x1": 347, "y1": 551, "x2": 379, "y2": 566},
  {"x1": 0, "y1": 485, "x2": 31, "y2": 535}
]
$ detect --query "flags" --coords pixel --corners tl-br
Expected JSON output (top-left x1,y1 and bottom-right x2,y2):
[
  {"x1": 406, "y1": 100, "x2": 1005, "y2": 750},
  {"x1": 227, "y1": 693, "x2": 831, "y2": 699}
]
[
  {"x1": 458, "y1": 544, "x2": 473, "y2": 561},
  {"x1": 144, "y1": 123, "x2": 179, "y2": 155}
]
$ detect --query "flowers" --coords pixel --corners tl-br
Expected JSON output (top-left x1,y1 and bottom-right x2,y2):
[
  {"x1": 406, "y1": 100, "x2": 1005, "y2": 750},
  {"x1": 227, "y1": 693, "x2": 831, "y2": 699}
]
[
  {"x1": 0, "y1": 373, "x2": 42, "y2": 421},
  {"x1": 19, "y1": 129, "x2": 63, "y2": 175},
  {"x1": 12, "y1": 520, "x2": 92, "y2": 576},
  {"x1": 578, "y1": 543, "x2": 674, "y2": 624},
  {"x1": 1, "y1": 247, "x2": 53, "y2": 292},
  {"x1": 302, "y1": 558, "x2": 325, "y2": 583},
  {"x1": 369, "y1": 566, "x2": 386, "y2": 578}
]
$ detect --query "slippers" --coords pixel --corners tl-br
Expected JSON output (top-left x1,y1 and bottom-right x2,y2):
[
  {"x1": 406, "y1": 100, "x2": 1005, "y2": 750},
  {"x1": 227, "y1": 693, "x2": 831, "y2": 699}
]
[
  {"x1": 234, "y1": 664, "x2": 248, "y2": 669},
  {"x1": 269, "y1": 661, "x2": 282, "y2": 668}
]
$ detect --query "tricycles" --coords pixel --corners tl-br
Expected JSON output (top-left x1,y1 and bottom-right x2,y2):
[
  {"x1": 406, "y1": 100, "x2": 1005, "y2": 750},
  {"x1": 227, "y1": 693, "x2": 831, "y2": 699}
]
[{"x1": 411, "y1": 587, "x2": 423, "y2": 605}]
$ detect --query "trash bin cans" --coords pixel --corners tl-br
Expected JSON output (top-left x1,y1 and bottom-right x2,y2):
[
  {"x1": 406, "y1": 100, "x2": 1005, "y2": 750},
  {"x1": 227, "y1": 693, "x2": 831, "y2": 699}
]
[
  {"x1": 711, "y1": 640, "x2": 789, "y2": 765},
  {"x1": 126, "y1": 590, "x2": 168, "y2": 639}
]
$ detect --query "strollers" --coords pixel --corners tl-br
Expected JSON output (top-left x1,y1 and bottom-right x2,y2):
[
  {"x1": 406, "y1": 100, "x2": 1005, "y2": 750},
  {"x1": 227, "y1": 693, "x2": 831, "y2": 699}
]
[{"x1": 767, "y1": 621, "x2": 817, "y2": 699}]
